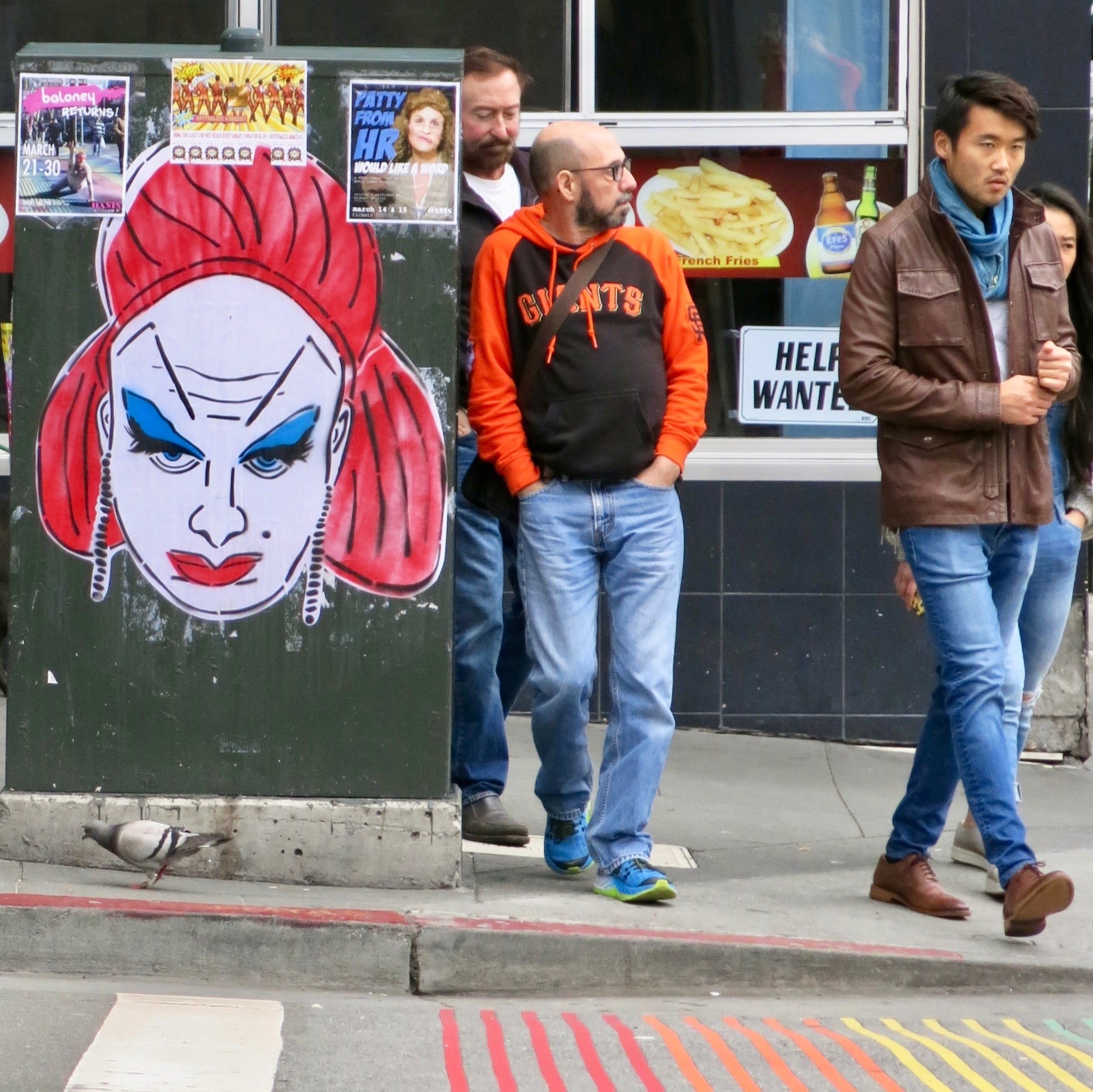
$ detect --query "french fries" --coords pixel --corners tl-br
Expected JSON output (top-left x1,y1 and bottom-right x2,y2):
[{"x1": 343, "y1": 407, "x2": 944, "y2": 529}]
[{"x1": 648, "y1": 157, "x2": 783, "y2": 258}]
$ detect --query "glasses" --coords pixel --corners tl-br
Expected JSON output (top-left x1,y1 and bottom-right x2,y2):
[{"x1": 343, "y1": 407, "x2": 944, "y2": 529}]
[{"x1": 556, "y1": 158, "x2": 632, "y2": 192}]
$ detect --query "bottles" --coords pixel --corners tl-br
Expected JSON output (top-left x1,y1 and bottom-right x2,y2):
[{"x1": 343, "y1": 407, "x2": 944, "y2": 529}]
[
  {"x1": 855, "y1": 162, "x2": 880, "y2": 250},
  {"x1": 813, "y1": 171, "x2": 857, "y2": 274}
]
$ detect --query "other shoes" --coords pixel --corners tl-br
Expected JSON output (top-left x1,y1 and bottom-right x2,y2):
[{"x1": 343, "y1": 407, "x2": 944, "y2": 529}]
[
  {"x1": 985, "y1": 864, "x2": 1005, "y2": 896},
  {"x1": 951, "y1": 820, "x2": 991, "y2": 871}
]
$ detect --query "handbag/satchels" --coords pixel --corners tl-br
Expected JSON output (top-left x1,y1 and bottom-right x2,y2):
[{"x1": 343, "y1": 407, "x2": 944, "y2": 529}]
[{"x1": 461, "y1": 452, "x2": 520, "y2": 527}]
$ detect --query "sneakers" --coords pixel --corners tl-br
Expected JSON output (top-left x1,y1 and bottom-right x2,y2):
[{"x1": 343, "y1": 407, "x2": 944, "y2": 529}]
[
  {"x1": 544, "y1": 811, "x2": 594, "y2": 874},
  {"x1": 592, "y1": 858, "x2": 676, "y2": 902}
]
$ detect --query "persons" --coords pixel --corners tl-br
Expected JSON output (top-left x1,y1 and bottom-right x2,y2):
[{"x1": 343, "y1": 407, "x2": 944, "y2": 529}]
[
  {"x1": 839, "y1": 70, "x2": 1081, "y2": 936},
  {"x1": 361, "y1": 87, "x2": 456, "y2": 221},
  {"x1": 894, "y1": 182, "x2": 1093, "y2": 895},
  {"x1": 451, "y1": 48, "x2": 534, "y2": 845},
  {"x1": 466, "y1": 120, "x2": 707, "y2": 902},
  {"x1": 21, "y1": 105, "x2": 125, "y2": 203}
]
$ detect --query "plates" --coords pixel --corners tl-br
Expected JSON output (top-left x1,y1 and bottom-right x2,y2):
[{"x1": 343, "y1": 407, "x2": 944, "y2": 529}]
[
  {"x1": 623, "y1": 202, "x2": 635, "y2": 226},
  {"x1": 805, "y1": 199, "x2": 893, "y2": 279},
  {"x1": 635, "y1": 166, "x2": 793, "y2": 257}
]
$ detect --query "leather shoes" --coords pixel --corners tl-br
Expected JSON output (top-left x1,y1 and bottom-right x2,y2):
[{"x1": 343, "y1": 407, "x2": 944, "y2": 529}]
[
  {"x1": 1003, "y1": 861, "x2": 1074, "y2": 937},
  {"x1": 461, "y1": 795, "x2": 530, "y2": 843},
  {"x1": 870, "y1": 853, "x2": 971, "y2": 916}
]
